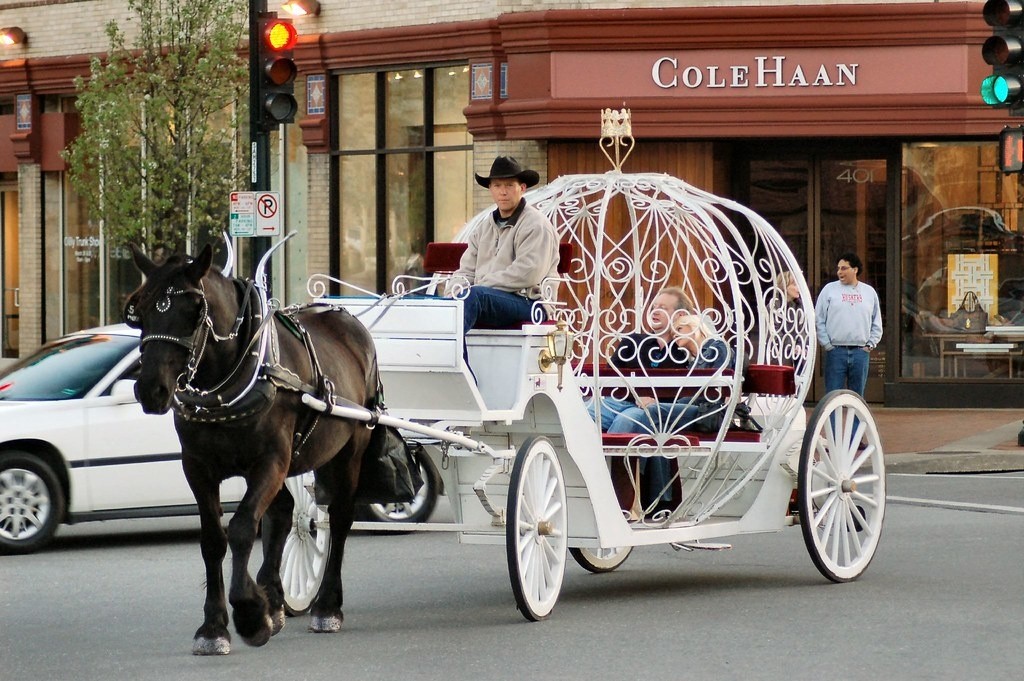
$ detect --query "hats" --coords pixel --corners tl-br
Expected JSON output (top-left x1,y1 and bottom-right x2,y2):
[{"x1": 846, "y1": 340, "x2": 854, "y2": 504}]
[{"x1": 475, "y1": 156, "x2": 540, "y2": 188}]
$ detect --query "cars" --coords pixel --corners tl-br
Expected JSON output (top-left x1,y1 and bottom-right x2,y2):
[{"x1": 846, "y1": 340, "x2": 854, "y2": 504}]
[{"x1": 0, "y1": 320, "x2": 456, "y2": 555}]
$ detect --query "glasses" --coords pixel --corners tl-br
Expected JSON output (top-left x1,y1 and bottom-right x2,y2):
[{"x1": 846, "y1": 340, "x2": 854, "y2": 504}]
[{"x1": 837, "y1": 266, "x2": 852, "y2": 271}]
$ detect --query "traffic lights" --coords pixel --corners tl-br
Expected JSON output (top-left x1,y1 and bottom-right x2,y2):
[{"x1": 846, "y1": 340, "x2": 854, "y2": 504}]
[
  {"x1": 979, "y1": 0, "x2": 1024, "y2": 111},
  {"x1": 257, "y1": 17, "x2": 299, "y2": 124}
]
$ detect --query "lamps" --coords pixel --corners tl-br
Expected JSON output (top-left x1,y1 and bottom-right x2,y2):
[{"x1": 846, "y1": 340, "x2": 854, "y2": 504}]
[
  {"x1": 0, "y1": 27, "x2": 28, "y2": 46},
  {"x1": 281, "y1": 0, "x2": 321, "y2": 17}
]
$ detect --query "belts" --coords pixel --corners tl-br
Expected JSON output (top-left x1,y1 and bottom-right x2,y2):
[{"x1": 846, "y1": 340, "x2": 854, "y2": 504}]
[{"x1": 838, "y1": 346, "x2": 861, "y2": 349}]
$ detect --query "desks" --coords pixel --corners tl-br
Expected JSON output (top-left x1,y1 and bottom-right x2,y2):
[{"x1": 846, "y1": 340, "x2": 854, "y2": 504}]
[{"x1": 922, "y1": 334, "x2": 1024, "y2": 379}]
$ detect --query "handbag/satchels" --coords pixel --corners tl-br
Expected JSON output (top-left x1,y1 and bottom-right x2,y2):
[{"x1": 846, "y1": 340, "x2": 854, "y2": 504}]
[{"x1": 694, "y1": 340, "x2": 764, "y2": 433}]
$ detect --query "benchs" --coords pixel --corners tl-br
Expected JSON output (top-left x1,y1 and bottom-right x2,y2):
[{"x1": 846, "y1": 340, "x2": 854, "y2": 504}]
[
  {"x1": 424, "y1": 242, "x2": 574, "y2": 337},
  {"x1": 602, "y1": 431, "x2": 713, "y2": 458},
  {"x1": 942, "y1": 349, "x2": 1023, "y2": 357},
  {"x1": 571, "y1": 362, "x2": 795, "y2": 453}
]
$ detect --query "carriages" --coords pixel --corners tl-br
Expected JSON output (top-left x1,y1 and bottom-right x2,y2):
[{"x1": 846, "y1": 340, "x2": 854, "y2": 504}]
[{"x1": 120, "y1": 105, "x2": 889, "y2": 659}]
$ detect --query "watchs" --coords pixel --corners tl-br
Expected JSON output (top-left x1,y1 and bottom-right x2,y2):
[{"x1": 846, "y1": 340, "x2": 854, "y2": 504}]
[{"x1": 866, "y1": 345, "x2": 873, "y2": 350}]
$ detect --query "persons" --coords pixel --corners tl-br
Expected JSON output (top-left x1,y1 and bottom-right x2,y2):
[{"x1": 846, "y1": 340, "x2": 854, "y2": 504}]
[
  {"x1": 585, "y1": 287, "x2": 736, "y2": 518},
  {"x1": 766, "y1": 252, "x2": 884, "y2": 452},
  {"x1": 443, "y1": 155, "x2": 561, "y2": 386}
]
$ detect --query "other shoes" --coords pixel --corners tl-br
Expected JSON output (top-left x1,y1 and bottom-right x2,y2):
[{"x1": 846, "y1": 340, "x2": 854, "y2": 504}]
[{"x1": 857, "y1": 442, "x2": 867, "y2": 450}]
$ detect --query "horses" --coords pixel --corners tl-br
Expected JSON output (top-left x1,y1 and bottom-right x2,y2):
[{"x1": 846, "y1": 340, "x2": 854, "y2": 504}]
[{"x1": 119, "y1": 226, "x2": 382, "y2": 658}]
